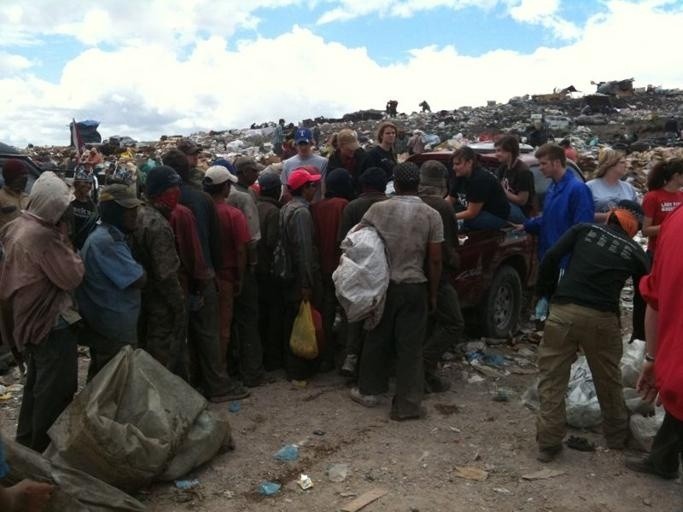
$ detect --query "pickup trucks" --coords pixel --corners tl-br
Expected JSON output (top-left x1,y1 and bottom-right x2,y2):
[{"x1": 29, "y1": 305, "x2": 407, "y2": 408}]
[{"x1": 382, "y1": 139, "x2": 588, "y2": 339}]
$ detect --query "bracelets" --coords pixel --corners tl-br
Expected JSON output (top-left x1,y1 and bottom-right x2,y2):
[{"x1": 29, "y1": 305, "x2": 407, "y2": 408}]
[{"x1": 643, "y1": 354, "x2": 655, "y2": 362}]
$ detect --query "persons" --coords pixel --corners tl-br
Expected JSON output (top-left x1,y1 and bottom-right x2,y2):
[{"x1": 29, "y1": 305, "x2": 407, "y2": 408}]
[
  {"x1": 641, "y1": 156, "x2": 683, "y2": 256},
  {"x1": 0, "y1": 441, "x2": 61, "y2": 511},
  {"x1": 623, "y1": 203, "x2": 683, "y2": 479},
  {"x1": 388, "y1": 100, "x2": 397, "y2": 117},
  {"x1": 535, "y1": 198, "x2": 652, "y2": 461},
  {"x1": 0, "y1": 117, "x2": 578, "y2": 454},
  {"x1": 509, "y1": 143, "x2": 595, "y2": 331},
  {"x1": 419, "y1": 100, "x2": 431, "y2": 112},
  {"x1": 585, "y1": 148, "x2": 640, "y2": 228}
]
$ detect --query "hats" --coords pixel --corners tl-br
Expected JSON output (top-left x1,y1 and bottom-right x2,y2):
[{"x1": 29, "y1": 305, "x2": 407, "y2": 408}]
[
  {"x1": 144, "y1": 164, "x2": 181, "y2": 198},
  {"x1": 337, "y1": 129, "x2": 362, "y2": 152},
  {"x1": 387, "y1": 160, "x2": 420, "y2": 185},
  {"x1": 294, "y1": 126, "x2": 312, "y2": 147},
  {"x1": 324, "y1": 167, "x2": 353, "y2": 196},
  {"x1": 230, "y1": 156, "x2": 266, "y2": 173},
  {"x1": 175, "y1": 139, "x2": 202, "y2": 154},
  {"x1": 618, "y1": 199, "x2": 645, "y2": 223},
  {"x1": 256, "y1": 171, "x2": 283, "y2": 192},
  {"x1": 285, "y1": 168, "x2": 320, "y2": 192},
  {"x1": 202, "y1": 159, "x2": 239, "y2": 189},
  {"x1": 96, "y1": 183, "x2": 146, "y2": 208},
  {"x1": 357, "y1": 166, "x2": 387, "y2": 191}
]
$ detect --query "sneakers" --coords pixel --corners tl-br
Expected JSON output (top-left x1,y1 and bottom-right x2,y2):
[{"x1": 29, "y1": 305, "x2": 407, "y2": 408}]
[
  {"x1": 536, "y1": 444, "x2": 563, "y2": 463},
  {"x1": 241, "y1": 369, "x2": 278, "y2": 387},
  {"x1": 626, "y1": 455, "x2": 660, "y2": 474},
  {"x1": 207, "y1": 383, "x2": 249, "y2": 405},
  {"x1": 389, "y1": 396, "x2": 428, "y2": 420},
  {"x1": 349, "y1": 385, "x2": 380, "y2": 408}
]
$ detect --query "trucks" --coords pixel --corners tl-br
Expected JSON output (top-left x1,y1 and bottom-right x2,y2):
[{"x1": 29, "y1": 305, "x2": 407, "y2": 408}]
[{"x1": 0, "y1": 139, "x2": 99, "y2": 228}]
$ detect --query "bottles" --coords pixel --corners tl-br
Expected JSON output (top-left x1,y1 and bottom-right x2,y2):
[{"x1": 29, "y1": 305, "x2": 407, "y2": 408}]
[{"x1": 458, "y1": 219, "x2": 466, "y2": 238}]
